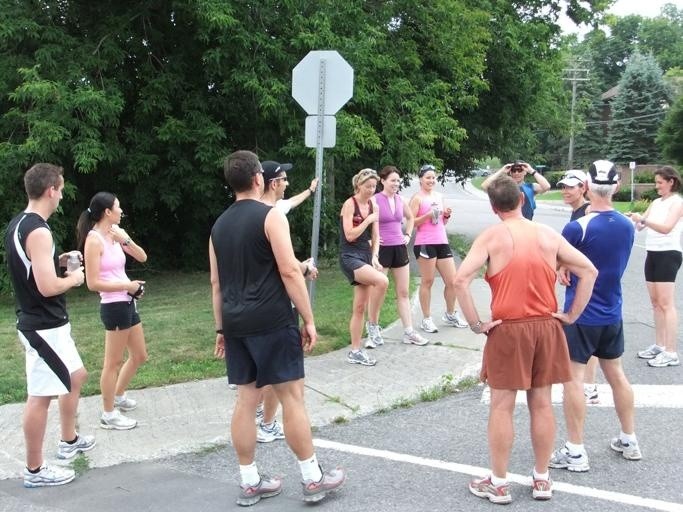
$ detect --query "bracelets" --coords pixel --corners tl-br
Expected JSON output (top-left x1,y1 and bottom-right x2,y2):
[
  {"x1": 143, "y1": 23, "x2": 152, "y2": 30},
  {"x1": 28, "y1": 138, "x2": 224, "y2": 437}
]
[
  {"x1": 307, "y1": 188, "x2": 315, "y2": 196},
  {"x1": 444, "y1": 215, "x2": 450, "y2": 219},
  {"x1": 532, "y1": 171, "x2": 537, "y2": 175},
  {"x1": 214, "y1": 328, "x2": 224, "y2": 335},
  {"x1": 471, "y1": 321, "x2": 482, "y2": 330},
  {"x1": 302, "y1": 264, "x2": 312, "y2": 276}
]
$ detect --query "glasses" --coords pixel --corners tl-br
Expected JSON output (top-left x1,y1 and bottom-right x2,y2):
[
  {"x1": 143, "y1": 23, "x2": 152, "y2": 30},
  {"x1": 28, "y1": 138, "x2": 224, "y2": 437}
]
[
  {"x1": 510, "y1": 168, "x2": 522, "y2": 172},
  {"x1": 356, "y1": 170, "x2": 376, "y2": 183},
  {"x1": 560, "y1": 174, "x2": 584, "y2": 184},
  {"x1": 418, "y1": 165, "x2": 435, "y2": 176},
  {"x1": 267, "y1": 176, "x2": 288, "y2": 184}
]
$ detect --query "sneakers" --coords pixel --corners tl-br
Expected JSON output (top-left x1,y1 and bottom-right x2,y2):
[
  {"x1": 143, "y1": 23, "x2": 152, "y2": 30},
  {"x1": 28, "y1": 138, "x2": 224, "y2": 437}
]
[
  {"x1": 468, "y1": 476, "x2": 511, "y2": 504},
  {"x1": 531, "y1": 475, "x2": 553, "y2": 500},
  {"x1": 610, "y1": 438, "x2": 640, "y2": 460},
  {"x1": 584, "y1": 385, "x2": 600, "y2": 405},
  {"x1": 548, "y1": 447, "x2": 589, "y2": 472},
  {"x1": 22, "y1": 459, "x2": 75, "y2": 488},
  {"x1": 99, "y1": 410, "x2": 137, "y2": 430},
  {"x1": 637, "y1": 345, "x2": 665, "y2": 359},
  {"x1": 509, "y1": 500, "x2": 511, "y2": 502},
  {"x1": 646, "y1": 352, "x2": 679, "y2": 367},
  {"x1": 228, "y1": 382, "x2": 346, "y2": 507},
  {"x1": 345, "y1": 310, "x2": 468, "y2": 366},
  {"x1": 55, "y1": 430, "x2": 96, "y2": 459},
  {"x1": 113, "y1": 394, "x2": 137, "y2": 410}
]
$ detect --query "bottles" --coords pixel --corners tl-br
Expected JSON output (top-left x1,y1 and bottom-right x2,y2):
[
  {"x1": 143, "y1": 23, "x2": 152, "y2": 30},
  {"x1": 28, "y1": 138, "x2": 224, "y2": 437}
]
[
  {"x1": 431, "y1": 202, "x2": 439, "y2": 226},
  {"x1": 64, "y1": 252, "x2": 84, "y2": 287}
]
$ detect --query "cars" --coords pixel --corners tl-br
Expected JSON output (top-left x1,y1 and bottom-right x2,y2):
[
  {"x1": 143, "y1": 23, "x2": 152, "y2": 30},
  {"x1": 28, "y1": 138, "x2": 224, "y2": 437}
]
[{"x1": 466, "y1": 167, "x2": 491, "y2": 177}]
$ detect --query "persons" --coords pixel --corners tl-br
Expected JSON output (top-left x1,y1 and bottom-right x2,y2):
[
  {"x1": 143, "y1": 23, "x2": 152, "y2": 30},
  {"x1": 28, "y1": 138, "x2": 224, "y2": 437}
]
[
  {"x1": 255, "y1": 161, "x2": 319, "y2": 442},
  {"x1": 481, "y1": 161, "x2": 551, "y2": 220},
  {"x1": 3, "y1": 163, "x2": 96, "y2": 486},
  {"x1": 626, "y1": 166, "x2": 683, "y2": 367},
  {"x1": 408, "y1": 166, "x2": 467, "y2": 332},
  {"x1": 75, "y1": 191, "x2": 148, "y2": 430},
  {"x1": 337, "y1": 166, "x2": 390, "y2": 366},
  {"x1": 555, "y1": 170, "x2": 598, "y2": 403},
  {"x1": 547, "y1": 160, "x2": 642, "y2": 472},
  {"x1": 365, "y1": 166, "x2": 429, "y2": 348},
  {"x1": 453, "y1": 177, "x2": 598, "y2": 502},
  {"x1": 206, "y1": 151, "x2": 347, "y2": 506}
]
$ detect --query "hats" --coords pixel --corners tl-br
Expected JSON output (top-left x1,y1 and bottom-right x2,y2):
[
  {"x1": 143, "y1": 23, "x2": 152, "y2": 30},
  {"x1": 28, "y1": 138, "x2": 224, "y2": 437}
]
[
  {"x1": 587, "y1": 160, "x2": 616, "y2": 185},
  {"x1": 555, "y1": 170, "x2": 586, "y2": 187},
  {"x1": 260, "y1": 160, "x2": 292, "y2": 183},
  {"x1": 512, "y1": 160, "x2": 524, "y2": 166}
]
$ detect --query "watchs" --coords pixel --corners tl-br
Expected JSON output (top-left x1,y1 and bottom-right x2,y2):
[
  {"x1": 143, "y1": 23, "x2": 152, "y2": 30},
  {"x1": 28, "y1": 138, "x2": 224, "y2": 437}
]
[
  {"x1": 122, "y1": 238, "x2": 131, "y2": 246},
  {"x1": 403, "y1": 231, "x2": 412, "y2": 238}
]
